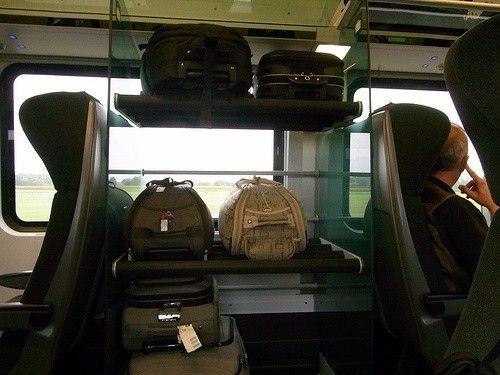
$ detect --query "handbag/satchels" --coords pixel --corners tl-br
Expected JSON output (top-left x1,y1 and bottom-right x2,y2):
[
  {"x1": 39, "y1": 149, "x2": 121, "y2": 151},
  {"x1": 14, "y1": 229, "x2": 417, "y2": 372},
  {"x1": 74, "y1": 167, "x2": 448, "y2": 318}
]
[
  {"x1": 125, "y1": 179, "x2": 216, "y2": 259},
  {"x1": 130, "y1": 316, "x2": 249, "y2": 375},
  {"x1": 218, "y1": 179, "x2": 309, "y2": 262}
]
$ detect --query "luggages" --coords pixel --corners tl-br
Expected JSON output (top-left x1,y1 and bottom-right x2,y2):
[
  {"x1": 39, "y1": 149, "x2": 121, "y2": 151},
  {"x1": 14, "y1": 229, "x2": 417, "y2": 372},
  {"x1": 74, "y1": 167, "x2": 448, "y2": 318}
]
[
  {"x1": 256, "y1": 49, "x2": 344, "y2": 101},
  {"x1": 122, "y1": 275, "x2": 221, "y2": 348},
  {"x1": 139, "y1": 21, "x2": 253, "y2": 102}
]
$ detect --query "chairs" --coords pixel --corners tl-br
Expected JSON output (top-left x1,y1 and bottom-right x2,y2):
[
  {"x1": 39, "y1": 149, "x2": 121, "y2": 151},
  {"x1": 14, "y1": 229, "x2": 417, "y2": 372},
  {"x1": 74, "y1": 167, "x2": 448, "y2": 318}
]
[
  {"x1": 0, "y1": 92, "x2": 101, "y2": 375},
  {"x1": 363, "y1": 14, "x2": 500, "y2": 375}
]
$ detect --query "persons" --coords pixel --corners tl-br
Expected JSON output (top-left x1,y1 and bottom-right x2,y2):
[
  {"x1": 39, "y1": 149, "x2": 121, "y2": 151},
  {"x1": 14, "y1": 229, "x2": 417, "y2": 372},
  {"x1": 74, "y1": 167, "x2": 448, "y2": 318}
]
[{"x1": 422, "y1": 124, "x2": 500, "y2": 338}]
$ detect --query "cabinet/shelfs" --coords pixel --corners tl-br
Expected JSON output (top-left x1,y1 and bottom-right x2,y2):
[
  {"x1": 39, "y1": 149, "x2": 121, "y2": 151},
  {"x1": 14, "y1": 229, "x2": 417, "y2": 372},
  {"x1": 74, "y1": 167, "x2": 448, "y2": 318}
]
[{"x1": 104, "y1": 0, "x2": 383, "y2": 375}]
